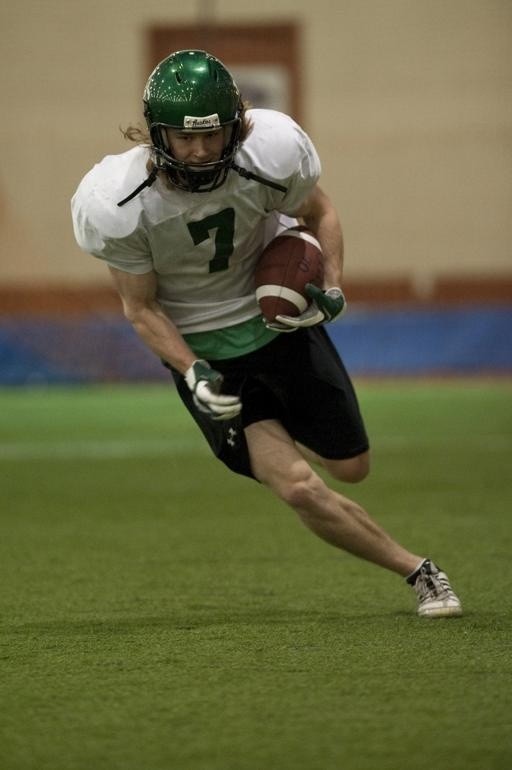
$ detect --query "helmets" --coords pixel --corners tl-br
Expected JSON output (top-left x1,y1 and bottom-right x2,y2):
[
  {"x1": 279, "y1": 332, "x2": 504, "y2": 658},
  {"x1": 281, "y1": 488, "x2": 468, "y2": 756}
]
[{"x1": 142, "y1": 49, "x2": 243, "y2": 147}]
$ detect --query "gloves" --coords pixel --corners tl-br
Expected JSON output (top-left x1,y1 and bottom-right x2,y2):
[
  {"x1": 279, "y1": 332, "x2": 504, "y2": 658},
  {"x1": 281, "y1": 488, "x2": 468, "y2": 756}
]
[
  {"x1": 185, "y1": 360, "x2": 242, "y2": 421},
  {"x1": 262, "y1": 283, "x2": 347, "y2": 333}
]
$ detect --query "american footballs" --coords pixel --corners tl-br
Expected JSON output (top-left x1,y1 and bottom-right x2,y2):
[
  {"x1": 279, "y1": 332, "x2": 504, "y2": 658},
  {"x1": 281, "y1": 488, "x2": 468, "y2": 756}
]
[{"x1": 257, "y1": 226, "x2": 325, "y2": 323}]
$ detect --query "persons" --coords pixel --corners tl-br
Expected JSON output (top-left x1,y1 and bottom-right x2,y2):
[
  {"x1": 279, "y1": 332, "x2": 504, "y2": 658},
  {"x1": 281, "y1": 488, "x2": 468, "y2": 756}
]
[{"x1": 70, "y1": 49, "x2": 463, "y2": 619}]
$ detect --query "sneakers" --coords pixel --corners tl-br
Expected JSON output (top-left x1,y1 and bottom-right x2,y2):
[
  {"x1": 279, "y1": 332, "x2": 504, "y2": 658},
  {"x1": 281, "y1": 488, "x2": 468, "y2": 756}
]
[{"x1": 405, "y1": 558, "x2": 463, "y2": 617}]
